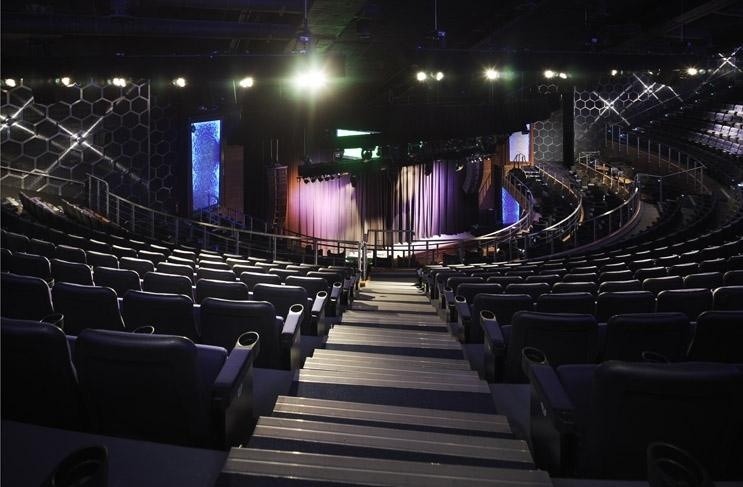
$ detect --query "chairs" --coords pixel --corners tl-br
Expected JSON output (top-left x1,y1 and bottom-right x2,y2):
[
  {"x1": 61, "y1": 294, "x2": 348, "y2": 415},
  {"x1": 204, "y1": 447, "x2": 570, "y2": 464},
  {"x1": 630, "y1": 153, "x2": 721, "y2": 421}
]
[
  {"x1": 0, "y1": 185, "x2": 364, "y2": 487},
  {"x1": 415, "y1": 97, "x2": 742, "y2": 486}
]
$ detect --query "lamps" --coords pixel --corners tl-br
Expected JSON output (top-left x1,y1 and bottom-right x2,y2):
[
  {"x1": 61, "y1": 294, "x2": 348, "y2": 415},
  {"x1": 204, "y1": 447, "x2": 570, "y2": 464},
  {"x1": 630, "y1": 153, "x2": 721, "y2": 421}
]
[{"x1": 298, "y1": 171, "x2": 350, "y2": 184}]
